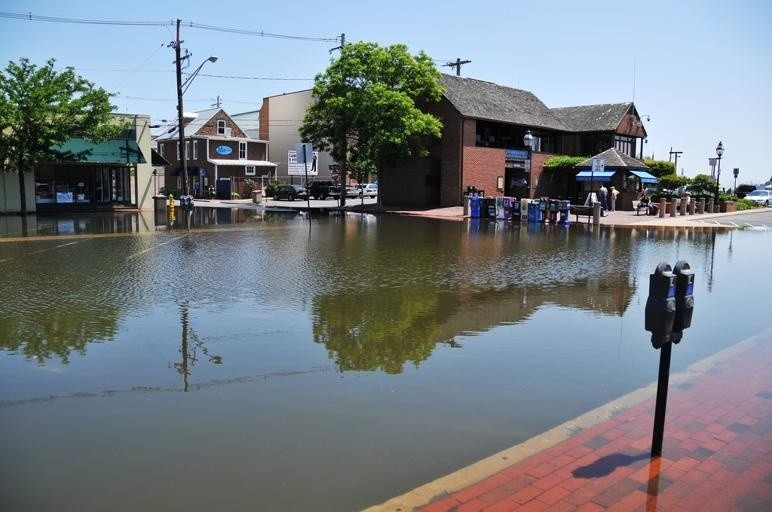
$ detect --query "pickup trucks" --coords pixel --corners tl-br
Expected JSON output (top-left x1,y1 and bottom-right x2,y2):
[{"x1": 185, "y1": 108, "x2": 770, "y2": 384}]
[{"x1": 309, "y1": 180, "x2": 342, "y2": 199}]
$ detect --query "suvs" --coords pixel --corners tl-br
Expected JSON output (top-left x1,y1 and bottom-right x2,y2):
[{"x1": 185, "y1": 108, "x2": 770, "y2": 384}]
[{"x1": 354, "y1": 184, "x2": 379, "y2": 198}]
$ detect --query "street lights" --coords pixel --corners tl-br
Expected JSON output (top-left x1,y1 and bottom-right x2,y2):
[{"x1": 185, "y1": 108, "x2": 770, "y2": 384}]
[
  {"x1": 733, "y1": 168, "x2": 740, "y2": 194},
  {"x1": 715, "y1": 141, "x2": 724, "y2": 204},
  {"x1": 176, "y1": 56, "x2": 218, "y2": 200},
  {"x1": 177, "y1": 299, "x2": 225, "y2": 392},
  {"x1": 524, "y1": 128, "x2": 533, "y2": 201}
]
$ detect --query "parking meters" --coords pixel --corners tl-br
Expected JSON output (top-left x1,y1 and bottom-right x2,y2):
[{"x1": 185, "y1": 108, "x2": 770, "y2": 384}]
[{"x1": 644, "y1": 257, "x2": 695, "y2": 456}]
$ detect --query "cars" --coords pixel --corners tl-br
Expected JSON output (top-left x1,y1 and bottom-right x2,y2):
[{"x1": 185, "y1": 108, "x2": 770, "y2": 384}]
[
  {"x1": 272, "y1": 185, "x2": 310, "y2": 201},
  {"x1": 744, "y1": 190, "x2": 772, "y2": 207},
  {"x1": 337, "y1": 183, "x2": 360, "y2": 199}
]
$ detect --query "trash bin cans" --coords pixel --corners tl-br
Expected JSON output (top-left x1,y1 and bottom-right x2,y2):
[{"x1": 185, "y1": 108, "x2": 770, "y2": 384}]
[
  {"x1": 252, "y1": 190, "x2": 262, "y2": 203},
  {"x1": 152, "y1": 195, "x2": 166, "y2": 212}
]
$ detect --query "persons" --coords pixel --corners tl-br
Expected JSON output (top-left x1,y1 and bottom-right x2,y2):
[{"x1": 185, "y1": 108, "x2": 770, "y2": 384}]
[
  {"x1": 596, "y1": 184, "x2": 608, "y2": 210},
  {"x1": 610, "y1": 186, "x2": 620, "y2": 210},
  {"x1": 645, "y1": 200, "x2": 658, "y2": 208},
  {"x1": 637, "y1": 187, "x2": 645, "y2": 203},
  {"x1": 310, "y1": 153, "x2": 318, "y2": 173}
]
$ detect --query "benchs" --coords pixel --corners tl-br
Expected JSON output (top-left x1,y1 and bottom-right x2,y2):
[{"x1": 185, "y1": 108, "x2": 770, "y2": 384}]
[
  {"x1": 632, "y1": 200, "x2": 650, "y2": 215},
  {"x1": 569, "y1": 203, "x2": 608, "y2": 224}
]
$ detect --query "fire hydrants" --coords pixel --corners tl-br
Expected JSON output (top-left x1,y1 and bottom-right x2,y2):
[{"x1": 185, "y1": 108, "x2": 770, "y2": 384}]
[
  {"x1": 169, "y1": 193, "x2": 175, "y2": 209},
  {"x1": 169, "y1": 208, "x2": 176, "y2": 226}
]
